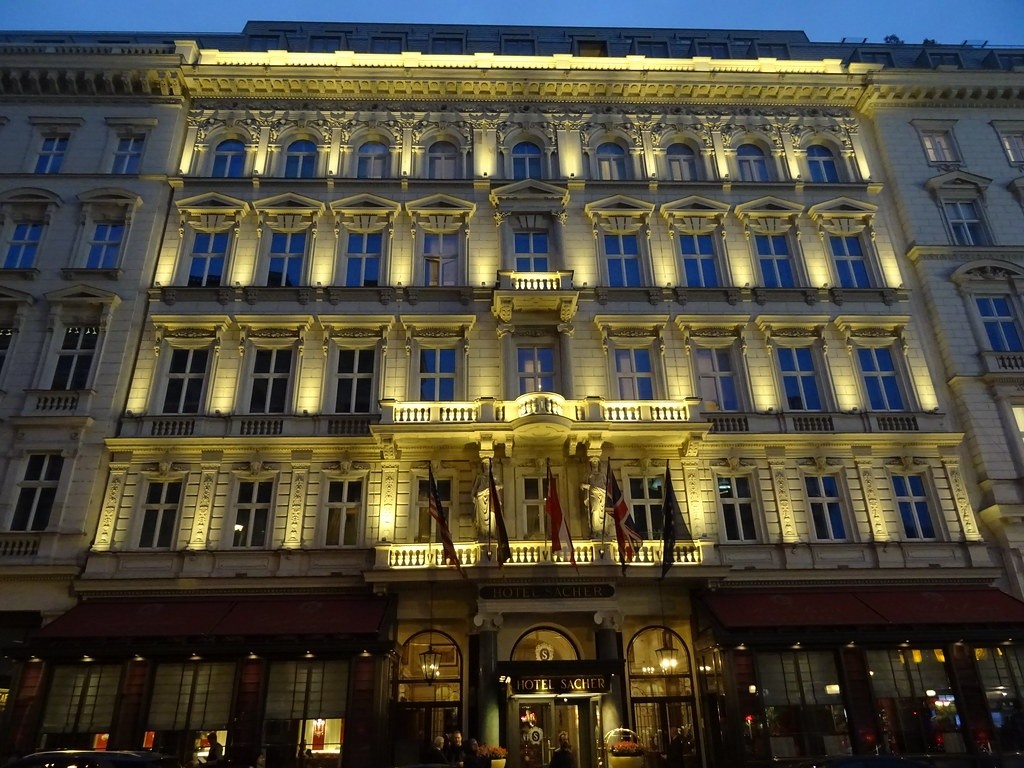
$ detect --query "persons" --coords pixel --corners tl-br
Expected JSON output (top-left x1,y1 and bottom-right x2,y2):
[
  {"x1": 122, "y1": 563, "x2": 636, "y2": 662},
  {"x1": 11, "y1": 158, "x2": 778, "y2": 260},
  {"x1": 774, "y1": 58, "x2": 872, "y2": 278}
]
[
  {"x1": 581, "y1": 458, "x2": 616, "y2": 538},
  {"x1": 471, "y1": 459, "x2": 504, "y2": 539},
  {"x1": 205, "y1": 733, "x2": 223, "y2": 768},
  {"x1": 549, "y1": 733, "x2": 576, "y2": 768},
  {"x1": 425, "y1": 731, "x2": 492, "y2": 768},
  {"x1": 660, "y1": 726, "x2": 682, "y2": 768}
]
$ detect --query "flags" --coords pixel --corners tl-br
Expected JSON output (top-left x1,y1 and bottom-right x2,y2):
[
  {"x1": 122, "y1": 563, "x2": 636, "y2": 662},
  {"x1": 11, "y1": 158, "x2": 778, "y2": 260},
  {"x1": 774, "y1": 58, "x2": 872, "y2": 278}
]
[
  {"x1": 661, "y1": 469, "x2": 696, "y2": 579},
  {"x1": 489, "y1": 470, "x2": 513, "y2": 570},
  {"x1": 546, "y1": 466, "x2": 580, "y2": 575},
  {"x1": 428, "y1": 471, "x2": 467, "y2": 580},
  {"x1": 605, "y1": 467, "x2": 643, "y2": 579}
]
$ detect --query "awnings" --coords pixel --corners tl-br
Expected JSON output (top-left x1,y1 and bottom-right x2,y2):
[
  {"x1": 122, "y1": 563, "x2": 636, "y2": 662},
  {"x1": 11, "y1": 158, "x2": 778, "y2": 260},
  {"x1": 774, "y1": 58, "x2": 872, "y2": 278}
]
[
  {"x1": 33, "y1": 596, "x2": 399, "y2": 654},
  {"x1": 696, "y1": 591, "x2": 1024, "y2": 628}
]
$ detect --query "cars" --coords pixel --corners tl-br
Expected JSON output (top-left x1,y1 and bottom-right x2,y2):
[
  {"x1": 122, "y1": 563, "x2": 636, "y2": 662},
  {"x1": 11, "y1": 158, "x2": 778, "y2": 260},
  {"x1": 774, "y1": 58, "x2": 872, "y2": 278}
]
[{"x1": 0, "y1": 750, "x2": 189, "y2": 767}]
